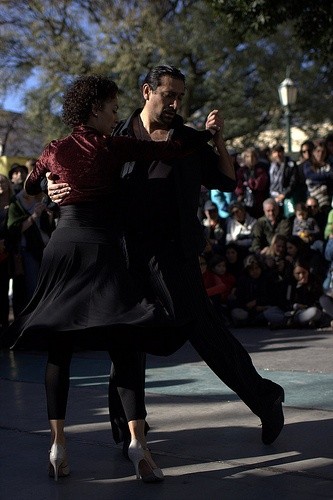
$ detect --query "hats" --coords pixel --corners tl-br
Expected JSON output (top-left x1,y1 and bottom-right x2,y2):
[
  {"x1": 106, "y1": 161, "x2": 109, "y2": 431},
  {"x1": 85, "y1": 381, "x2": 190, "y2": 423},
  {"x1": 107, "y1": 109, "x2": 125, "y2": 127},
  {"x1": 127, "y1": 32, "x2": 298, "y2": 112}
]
[
  {"x1": 229, "y1": 201, "x2": 242, "y2": 212},
  {"x1": 204, "y1": 202, "x2": 217, "y2": 210},
  {"x1": 207, "y1": 254, "x2": 224, "y2": 270},
  {"x1": 9, "y1": 163, "x2": 28, "y2": 180}
]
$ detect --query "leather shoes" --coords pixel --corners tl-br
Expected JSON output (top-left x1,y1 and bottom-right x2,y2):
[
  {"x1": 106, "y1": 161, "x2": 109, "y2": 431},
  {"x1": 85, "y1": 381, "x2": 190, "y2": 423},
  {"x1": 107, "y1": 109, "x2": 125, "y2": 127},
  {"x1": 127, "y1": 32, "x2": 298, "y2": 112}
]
[
  {"x1": 128, "y1": 439, "x2": 164, "y2": 481},
  {"x1": 261, "y1": 383, "x2": 284, "y2": 445},
  {"x1": 49, "y1": 443, "x2": 70, "y2": 481},
  {"x1": 122, "y1": 440, "x2": 133, "y2": 462}
]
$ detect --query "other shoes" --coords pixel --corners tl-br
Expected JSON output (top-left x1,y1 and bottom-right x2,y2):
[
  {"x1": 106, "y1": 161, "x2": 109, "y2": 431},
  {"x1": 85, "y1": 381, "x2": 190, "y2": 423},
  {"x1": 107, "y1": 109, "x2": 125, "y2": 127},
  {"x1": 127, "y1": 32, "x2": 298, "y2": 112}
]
[
  {"x1": 0, "y1": 323, "x2": 9, "y2": 328},
  {"x1": 273, "y1": 425, "x2": 275, "y2": 426},
  {"x1": 310, "y1": 319, "x2": 326, "y2": 329}
]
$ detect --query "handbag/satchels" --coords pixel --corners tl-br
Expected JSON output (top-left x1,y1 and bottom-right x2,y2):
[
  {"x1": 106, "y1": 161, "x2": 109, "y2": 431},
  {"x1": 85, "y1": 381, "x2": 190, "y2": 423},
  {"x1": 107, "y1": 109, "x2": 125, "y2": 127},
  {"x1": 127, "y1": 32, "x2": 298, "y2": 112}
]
[
  {"x1": 7, "y1": 239, "x2": 24, "y2": 278},
  {"x1": 241, "y1": 186, "x2": 254, "y2": 207},
  {"x1": 323, "y1": 262, "x2": 333, "y2": 297},
  {"x1": 0, "y1": 239, "x2": 11, "y2": 262}
]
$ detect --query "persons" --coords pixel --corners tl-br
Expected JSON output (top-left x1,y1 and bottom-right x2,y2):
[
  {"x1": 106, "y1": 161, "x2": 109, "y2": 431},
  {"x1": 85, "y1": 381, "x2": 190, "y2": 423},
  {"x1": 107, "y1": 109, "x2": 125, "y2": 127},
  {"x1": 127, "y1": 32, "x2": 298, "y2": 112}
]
[{"x1": 0, "y1": 63, "x2": 332, "y2": 481}]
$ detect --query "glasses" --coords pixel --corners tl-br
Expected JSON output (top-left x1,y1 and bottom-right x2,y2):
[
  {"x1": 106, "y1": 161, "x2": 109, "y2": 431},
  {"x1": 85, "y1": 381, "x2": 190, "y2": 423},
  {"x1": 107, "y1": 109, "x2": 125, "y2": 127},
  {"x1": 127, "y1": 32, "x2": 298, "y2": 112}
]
[
  {"x1": 307, "y1": 205, "x2": 317, "y2": 209},
  {"x1": 199, "y1": 262, "x2": 208, "y2": 266}
]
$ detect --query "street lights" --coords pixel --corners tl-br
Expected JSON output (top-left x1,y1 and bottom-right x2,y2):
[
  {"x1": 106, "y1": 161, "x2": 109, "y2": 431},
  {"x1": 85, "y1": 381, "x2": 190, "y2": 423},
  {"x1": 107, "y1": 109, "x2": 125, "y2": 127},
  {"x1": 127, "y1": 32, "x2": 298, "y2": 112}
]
[{"x1": 277, "y1": 76, "x2": 297, "y2": 157}]
